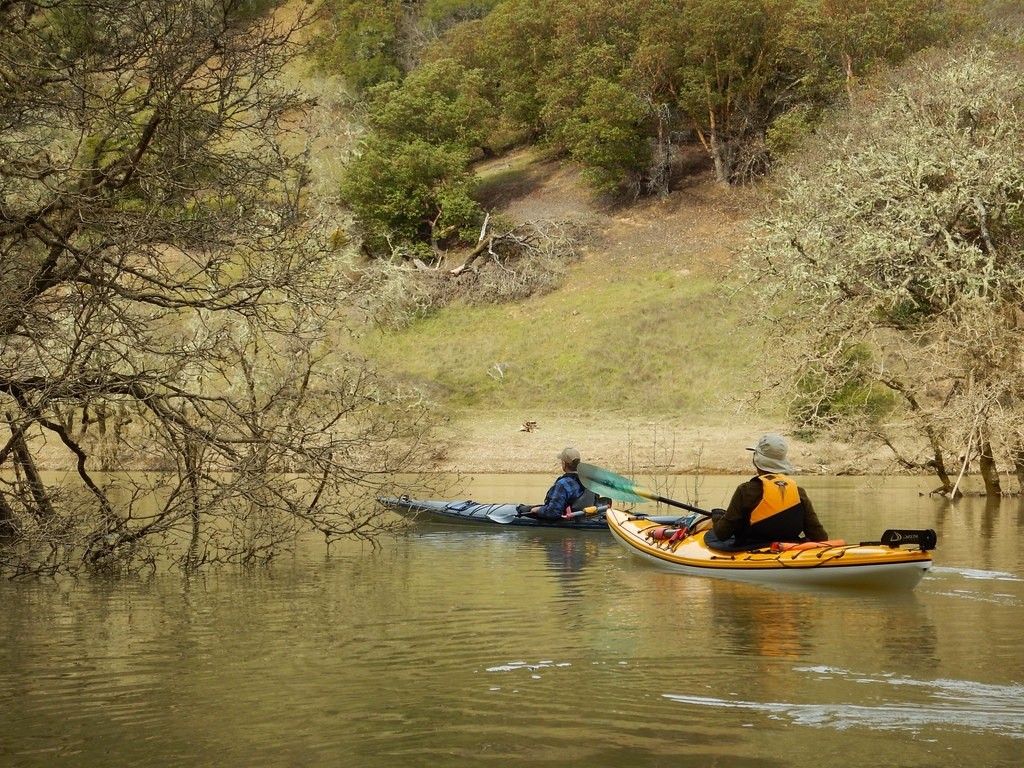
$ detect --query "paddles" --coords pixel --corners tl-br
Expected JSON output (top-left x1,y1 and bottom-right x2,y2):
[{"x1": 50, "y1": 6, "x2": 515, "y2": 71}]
[
  {"x1": 486, "y1": 510, "x2": 540, "y2": 523},
  {"x1": 574, "y1": 462, "x2": 712, "y2": 515}
]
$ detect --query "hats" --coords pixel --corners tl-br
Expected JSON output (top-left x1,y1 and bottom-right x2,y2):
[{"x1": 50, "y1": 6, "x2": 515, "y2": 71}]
[
  {"x1": 556, "y1": 448, "x2": 581, "y2": 466},
  {"x1": 746, "y1": 434, "x2": 794, "y2": 475}
]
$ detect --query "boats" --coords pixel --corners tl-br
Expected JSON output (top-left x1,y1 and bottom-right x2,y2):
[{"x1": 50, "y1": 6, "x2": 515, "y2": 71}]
[
  {"x1": 606, "y1": 508, "x2": 937, "y2": 589},
  {"x1": 375, "y1": 494, "x2": 684, "y2": 529}
]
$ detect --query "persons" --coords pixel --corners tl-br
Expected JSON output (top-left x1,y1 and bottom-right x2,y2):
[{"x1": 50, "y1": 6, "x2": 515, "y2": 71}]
[
  {"x1": 711, "y1": 434, "x2": 828, "y2": 551},
  {"x1": 516, "y1": 447, "x2": 600, "y2": 522}
]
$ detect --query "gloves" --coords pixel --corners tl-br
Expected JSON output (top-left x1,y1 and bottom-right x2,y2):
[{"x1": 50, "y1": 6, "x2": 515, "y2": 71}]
[{"x1": 711, "y1": 508, "x2": 726, "y2": 515}]
